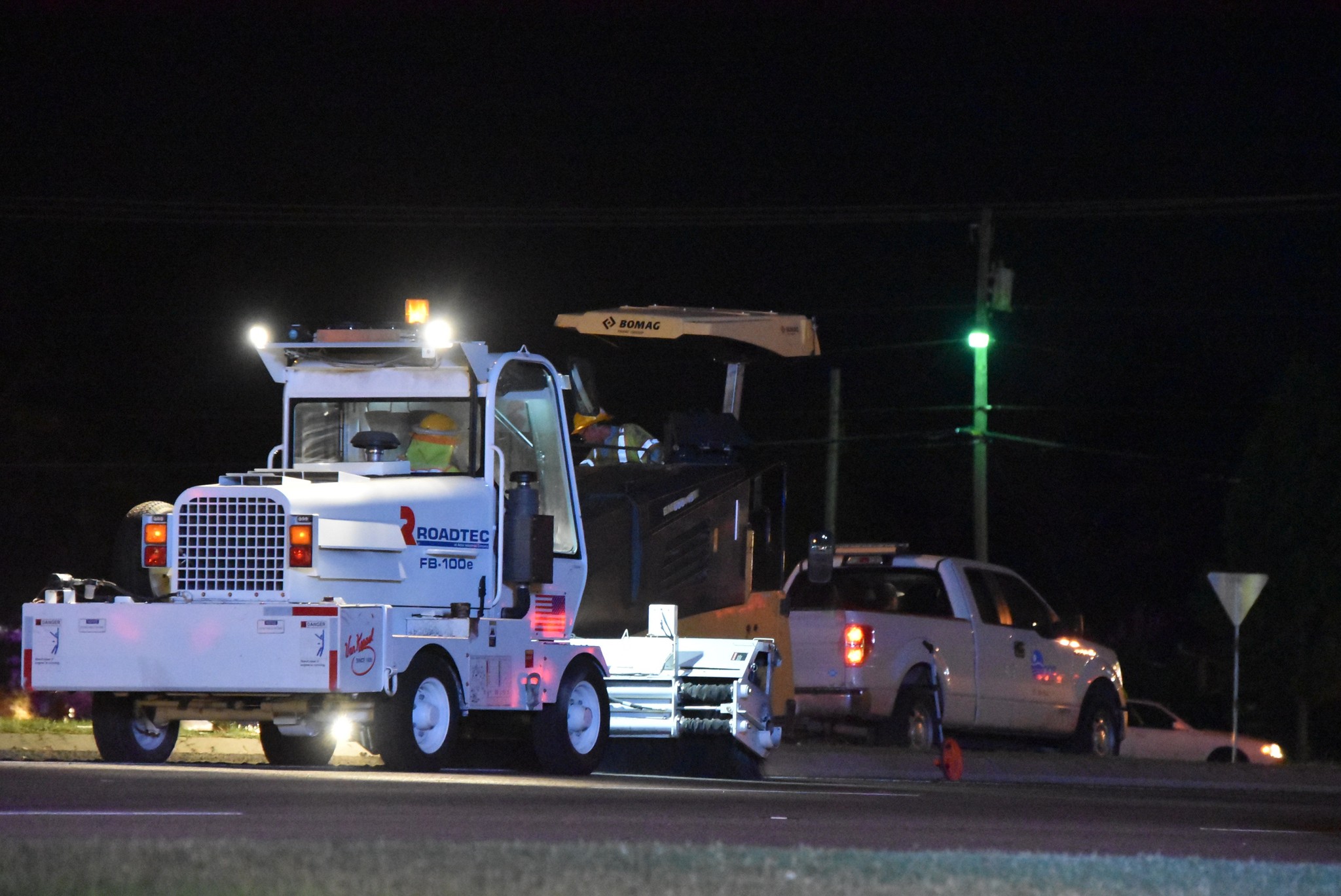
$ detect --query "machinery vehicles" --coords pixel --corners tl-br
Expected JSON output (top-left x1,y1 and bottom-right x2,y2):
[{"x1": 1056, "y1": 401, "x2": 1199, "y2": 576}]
[{"x1": 20, "y1": 300, "x2": 782, "y2": 776}]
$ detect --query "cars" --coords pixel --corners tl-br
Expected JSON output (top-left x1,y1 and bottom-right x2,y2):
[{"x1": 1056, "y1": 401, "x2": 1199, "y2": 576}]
[
  {"x1": 1118, "y1": 698, "x2": 1285, "y2": 765},
  {"x1": 784, "y1": 554, "x2": 1130, "y2": 759}
]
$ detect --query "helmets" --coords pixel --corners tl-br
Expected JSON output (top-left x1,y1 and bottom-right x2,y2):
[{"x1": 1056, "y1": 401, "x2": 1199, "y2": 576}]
[
  {"x1": 409, "y1": 412, "x2": 460, "y2": 445},
  {"x1": 570, "y1": 405, "x2": 614, "y2": 434}
]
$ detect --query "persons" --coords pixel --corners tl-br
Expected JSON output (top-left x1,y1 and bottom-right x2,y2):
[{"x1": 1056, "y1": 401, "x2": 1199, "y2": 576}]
[
  {"x1": 394, "y1": 413, "x2": 461, "y2": 473},
  {"x1": 571, "y1": 407, "x2": 661, "y2": 467}
]
